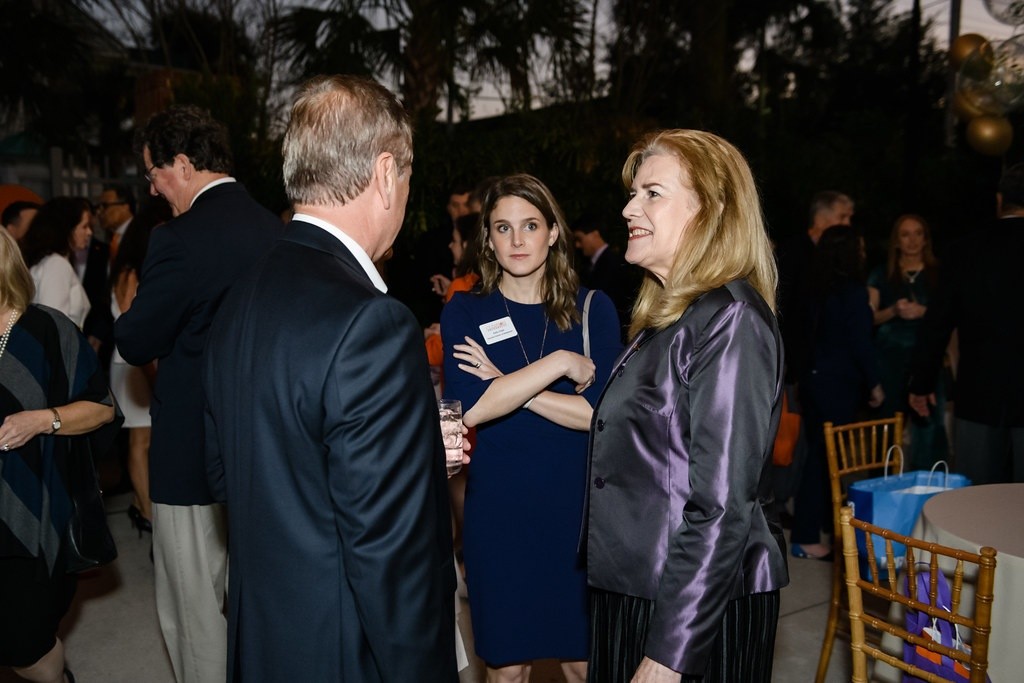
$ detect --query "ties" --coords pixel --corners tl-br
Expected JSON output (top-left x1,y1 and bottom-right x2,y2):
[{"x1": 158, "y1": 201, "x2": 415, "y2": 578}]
[{"x1": 110, "y1": 231, "x2": 120, "y2": 268}]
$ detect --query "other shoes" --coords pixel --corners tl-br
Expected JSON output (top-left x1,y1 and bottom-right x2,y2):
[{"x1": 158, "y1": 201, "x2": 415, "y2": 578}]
[{"x1": 791, "y1": 541, "x2": 836, "y2": 563}]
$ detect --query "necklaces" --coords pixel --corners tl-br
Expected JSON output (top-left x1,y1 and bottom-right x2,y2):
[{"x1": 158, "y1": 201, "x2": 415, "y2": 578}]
[
  {"x1": 0, "y1": 308, "x2": 16, "y2": 348},
  {"x1": 0, "y1": 310, "x2": 18, "y2": 358},
  {"x1": 504, "y1": 297, "x2": 549, "y2": 365}
]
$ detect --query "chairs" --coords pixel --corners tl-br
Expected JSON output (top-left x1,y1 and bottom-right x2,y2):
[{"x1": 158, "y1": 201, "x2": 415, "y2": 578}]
[{"x1": 815, "y1": 412, "x2": 996, "y2": 683}]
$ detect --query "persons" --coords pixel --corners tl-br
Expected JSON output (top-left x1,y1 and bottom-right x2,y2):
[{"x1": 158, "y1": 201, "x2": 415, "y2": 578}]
[
  {"x1": 441, "y1": 173, "x2": 621, "y2": 683},
  {"x1": 0, "y1": 181, "x2": 152, "y2": 539},
  {"x1": 114, "y1": 101, "x2": 285, "y2": 683},
  {"x1": 572, "y1": 214, "x2": 642, "y2": 346},
  {"x1": 785, "y1": 168, "x2": 1024, "y2": 561},
  {"x1": 424, "y1": 168, "x2": 483, "y2": 527},
  {"x1": 575, "y1": 129, "x2": 788, "y2": 683},
  {"x1": 199, "y1": 75, "x2": 458, "y2": 683},
  {"x1": 0, "y1": 225, "x2": 126, "y2": 683}
]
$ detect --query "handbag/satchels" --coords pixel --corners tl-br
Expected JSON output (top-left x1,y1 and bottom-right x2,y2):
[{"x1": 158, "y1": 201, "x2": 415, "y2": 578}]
[
  {"x1": 847, "y1": 444, "x2": 972, "y2": 583},
  {"x1": 899, "y1": 556, "x2": 993, "y2": 683},
  {"x1": 770, "y1": 389, "x2": 803, "y2": 466},
  {"x1": 58, "y1": 491, "x2": 116, "y2": 581}
]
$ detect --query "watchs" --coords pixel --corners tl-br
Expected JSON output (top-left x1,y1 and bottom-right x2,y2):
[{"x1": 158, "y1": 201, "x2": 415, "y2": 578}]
[{"x1": 49, "y1": 408, "x2": 62, "y2": 435}]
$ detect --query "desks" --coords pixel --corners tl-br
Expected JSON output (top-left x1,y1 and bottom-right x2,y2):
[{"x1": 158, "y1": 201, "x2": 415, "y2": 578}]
[{"x1": 874, "y1": 484, "x2": 1024, "y2": 683}]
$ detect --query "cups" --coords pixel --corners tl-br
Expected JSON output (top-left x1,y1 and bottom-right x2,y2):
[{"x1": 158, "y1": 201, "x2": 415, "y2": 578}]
[{"x1": 437, "y1": 398, "x2": 464, "y2": 474}]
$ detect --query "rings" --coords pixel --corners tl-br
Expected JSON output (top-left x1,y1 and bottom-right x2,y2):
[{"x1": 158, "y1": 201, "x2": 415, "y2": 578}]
[
  {"x1": 476, "y1": 363, "x2": 482, "y2": 368},
  {"x1": 5, "y1": 444, "x2": 10, "y2": 451}
]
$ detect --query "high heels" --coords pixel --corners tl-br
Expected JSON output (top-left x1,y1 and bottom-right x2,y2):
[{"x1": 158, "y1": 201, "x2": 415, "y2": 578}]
[{"x1": 127, "y1": 504, "x2": 153, "y2": 538}]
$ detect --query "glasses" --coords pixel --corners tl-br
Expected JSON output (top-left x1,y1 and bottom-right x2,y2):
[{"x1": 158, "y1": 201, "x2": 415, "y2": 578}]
[
  {"x1": 94, "y1": 199, "x2": 126, "y2": 213},
  {"x1": 144, "y1": 150, "x2": 196, "y2": 184}
]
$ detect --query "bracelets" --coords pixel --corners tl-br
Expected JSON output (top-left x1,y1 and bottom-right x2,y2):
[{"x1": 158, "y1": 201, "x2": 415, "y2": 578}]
[{"x1": 523, "y1": 397, "x2": 535, "y2": 409}]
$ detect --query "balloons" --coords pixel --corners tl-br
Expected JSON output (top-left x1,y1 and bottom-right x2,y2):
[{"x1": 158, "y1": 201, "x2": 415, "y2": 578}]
[{"x1": 951, "y1": 0, "x2": 1024, "y2": 156}]
